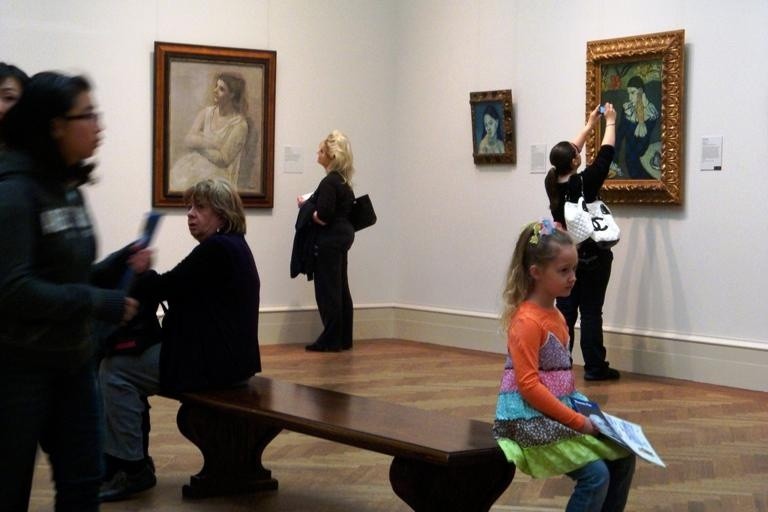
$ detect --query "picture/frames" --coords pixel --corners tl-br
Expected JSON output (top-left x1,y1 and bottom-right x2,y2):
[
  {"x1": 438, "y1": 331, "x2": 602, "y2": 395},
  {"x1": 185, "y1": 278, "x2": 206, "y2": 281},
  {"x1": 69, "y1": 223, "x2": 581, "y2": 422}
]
[
  {"x1": 153, "y1": 39, "x2": 276, "y2": 210},
  {"x1": 584, "y1": 30, "x2": 685, "y2": 204},
  {"x1": 468, "y1": 88, "x2": 516, "y2": 165}
]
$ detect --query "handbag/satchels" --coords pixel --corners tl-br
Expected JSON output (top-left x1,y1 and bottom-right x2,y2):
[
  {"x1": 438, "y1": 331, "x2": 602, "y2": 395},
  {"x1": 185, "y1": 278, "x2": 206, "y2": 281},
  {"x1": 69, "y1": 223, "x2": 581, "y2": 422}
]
[
  {"x1": 97, "y1": 269, "x2": 162, "y2": 357},
  {"x1": 564, "y1": 196, "x2": 621, "y2": 249},
  {"x1": 354, "y1": 194, "x2": 376, "y2": 231}
]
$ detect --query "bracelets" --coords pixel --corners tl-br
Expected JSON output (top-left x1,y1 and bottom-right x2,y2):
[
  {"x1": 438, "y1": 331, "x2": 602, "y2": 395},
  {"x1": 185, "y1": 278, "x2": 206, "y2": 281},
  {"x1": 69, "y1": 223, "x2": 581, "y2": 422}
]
[{"x1": 606, "y1": 122, "x2": 617, "y2": 126}]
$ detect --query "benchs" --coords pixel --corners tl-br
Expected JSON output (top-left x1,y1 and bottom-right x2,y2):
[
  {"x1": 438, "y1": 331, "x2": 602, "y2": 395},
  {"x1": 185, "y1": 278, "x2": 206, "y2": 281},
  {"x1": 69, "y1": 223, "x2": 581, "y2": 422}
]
[{"x1": 149, "y1": 374, "x2": 514, "y2": 511}]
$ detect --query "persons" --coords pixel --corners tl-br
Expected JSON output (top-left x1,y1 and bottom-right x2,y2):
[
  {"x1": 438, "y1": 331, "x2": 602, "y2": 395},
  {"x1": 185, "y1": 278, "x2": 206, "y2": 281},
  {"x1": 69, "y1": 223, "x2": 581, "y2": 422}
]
[
  {"x1": 1, "y1": 63, "x2": 32, "y2": 117},
  {"x1": 95, "y1": 179, "x2": 262, "y2": 501},
  {"x1": 167, "y1": 73, "x2": 252, "y2": 192},
  {"x1": 1, "y1": 69, "x2": 159, "y2": 512},
  {"x1": 544, "y1": 102, "x2": 623, "y2": 382},
  {"x1": 475, "y1": 105, "x2": 504, "y2": 154},
  {"x1": 608, "y1": 75, "x2": 661, "y2": 180},
  {"x1": 494, "y1": 217, "x2": 636, "y2": 512},
  {"x1": 289, "y1": 129, "x2": 359, "y2": 353}
]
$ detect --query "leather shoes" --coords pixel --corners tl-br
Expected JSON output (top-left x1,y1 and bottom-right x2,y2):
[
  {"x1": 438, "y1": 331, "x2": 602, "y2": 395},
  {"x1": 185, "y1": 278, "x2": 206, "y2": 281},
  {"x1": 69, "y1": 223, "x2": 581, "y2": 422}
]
[{"x1": 95, "y1": 465, "x2": 157, "y2": 503}]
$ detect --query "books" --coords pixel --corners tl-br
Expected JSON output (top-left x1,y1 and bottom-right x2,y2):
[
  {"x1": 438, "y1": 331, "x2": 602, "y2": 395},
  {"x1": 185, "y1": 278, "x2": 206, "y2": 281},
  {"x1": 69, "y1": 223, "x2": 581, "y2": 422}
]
[{"x1": 568, "y1": 396, "x2": 668, "y2": 471}]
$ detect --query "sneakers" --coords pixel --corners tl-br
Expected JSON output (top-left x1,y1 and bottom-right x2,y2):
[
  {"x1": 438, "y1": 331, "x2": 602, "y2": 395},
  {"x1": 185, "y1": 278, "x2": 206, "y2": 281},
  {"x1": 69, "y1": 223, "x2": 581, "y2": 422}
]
[{"x1": 584, "y1": 368, "x2": 619, "y2": 380}]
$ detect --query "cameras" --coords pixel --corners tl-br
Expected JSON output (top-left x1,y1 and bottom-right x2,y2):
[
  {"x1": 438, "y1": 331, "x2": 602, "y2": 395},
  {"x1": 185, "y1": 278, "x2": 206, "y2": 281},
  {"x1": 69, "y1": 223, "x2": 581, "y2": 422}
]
[{"x1": 599, "y1": 105, "x2": 606, "y2": 113}]
